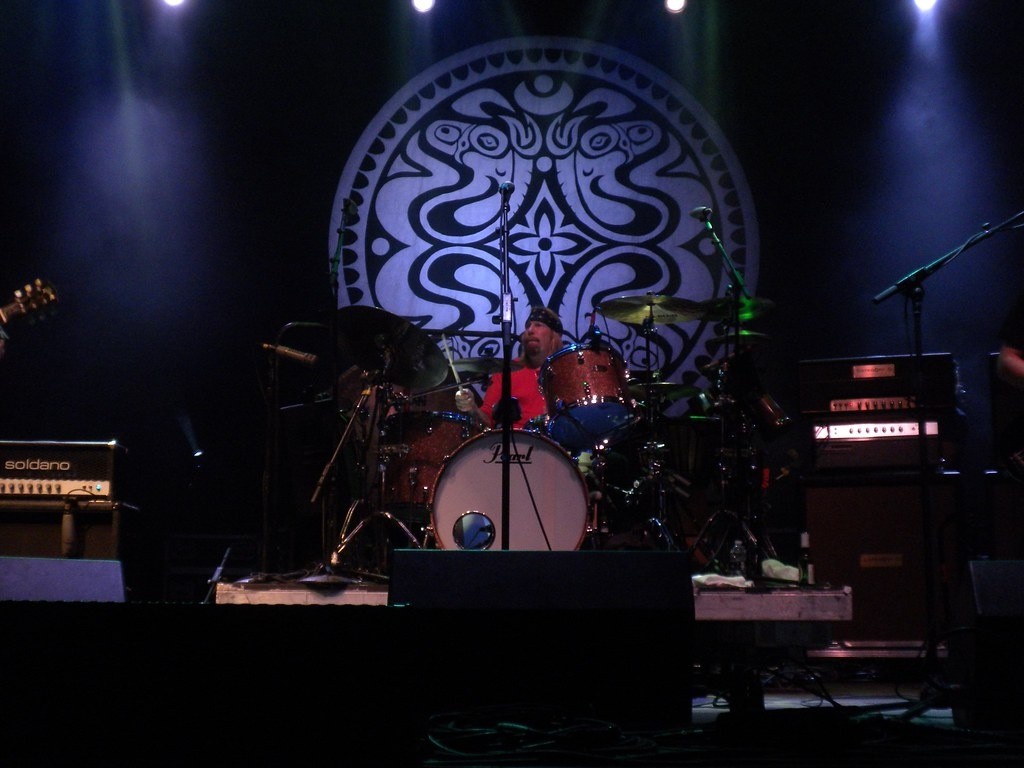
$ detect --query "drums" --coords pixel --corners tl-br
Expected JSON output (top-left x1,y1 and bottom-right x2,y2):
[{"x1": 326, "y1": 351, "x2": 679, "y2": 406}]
[
  {"x1": 380, "y1": 408, "x2": 477, "y2": 523},
  {"x1": 538, "y1": 343, "x2": 636, "y2": 449},
  {"x1": 427, "y1": 426, "x2": 591, "y2": 551}
]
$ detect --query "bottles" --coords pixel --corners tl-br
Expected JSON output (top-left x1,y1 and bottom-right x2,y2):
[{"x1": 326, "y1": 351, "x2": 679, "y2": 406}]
[
  {"x1": 799, "y1": 529, "x2": 816, "y2": 588},
  {"x1": 730, "y1": 540, "x2": 745, "y2": 577},
  {"x1": 747, "y1": 538, "x2": 762, "y2": 580}
]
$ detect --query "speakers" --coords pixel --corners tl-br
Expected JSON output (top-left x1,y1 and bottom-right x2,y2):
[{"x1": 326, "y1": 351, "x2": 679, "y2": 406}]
[
  {"x1": 0, "y1": 497, "x2": 144, "y2": 604},
  {"x1": 807, "y1": 469, "x2": 1013, "y2": 647},
  {"x1": 932, "y1": 558, "x2": 1024, "y2": 656}
]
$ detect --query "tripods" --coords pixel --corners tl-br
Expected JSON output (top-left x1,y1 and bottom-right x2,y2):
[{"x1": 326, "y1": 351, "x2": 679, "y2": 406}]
[
  {"x1": 597, "y1": 311, "x2": 778, "y2": 581},
  {"x1": 307, "y1": 350, "x2": 425, "y2": 576}
]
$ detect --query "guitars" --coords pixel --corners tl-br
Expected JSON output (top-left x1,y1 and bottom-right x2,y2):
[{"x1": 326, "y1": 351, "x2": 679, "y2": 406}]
[{"x1": 0, "y1": 277, "x2": 60, "y2": 327}]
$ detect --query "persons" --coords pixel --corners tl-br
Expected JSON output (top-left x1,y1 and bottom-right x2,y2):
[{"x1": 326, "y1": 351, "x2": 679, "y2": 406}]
[
  {"x1": 454, "y1": 307, "x2": 564, "y2": 430},
  {"x1": 997, "y1": 294, "x2": 1024, "y2": 391}
]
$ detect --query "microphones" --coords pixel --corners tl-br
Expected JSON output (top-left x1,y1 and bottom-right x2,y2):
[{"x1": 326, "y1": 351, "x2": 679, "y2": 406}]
[
  {"x1": 480, "y1": 524, "x2": 494, "y2": 533},
  {"x1": 260, "y1": 342, "x2": 319, "y2": 365},
  {"x1": 689, "y1": 205, "x2": 713, "y2": 220},
  {"x1": 703, "y1": 343, "x2": 758, "y2": 369},
  {"x1": 588, "y1": 309, "x2": 595, "y2": 339},
  {"x1": 500, "y1": 184, "x2": 516, "y2": 195},
  {"x1": 61, "y1": 499, "x2": 80, "y2": 559}
]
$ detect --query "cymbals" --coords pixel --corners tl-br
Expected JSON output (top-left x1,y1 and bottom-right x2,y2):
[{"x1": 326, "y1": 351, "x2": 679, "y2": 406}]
[
  {"x1": 695, "y1": 298, "x2": 777, "y2": 326},
  {"x1": 633, "y1": 382, "x2": 703, "y2": 400},
  {"x1": 450, "y1": 356, "x2": 526, "y2": 373},
  {"x1": 340, "y1": 304, "x2": 449, "y2": 392},
  {"x1": 714, "y1": 329, "x2": 768, "y2": 344},
  {"x1": 596, "y1": 295, "x2": 708, "y2": 326}
]
